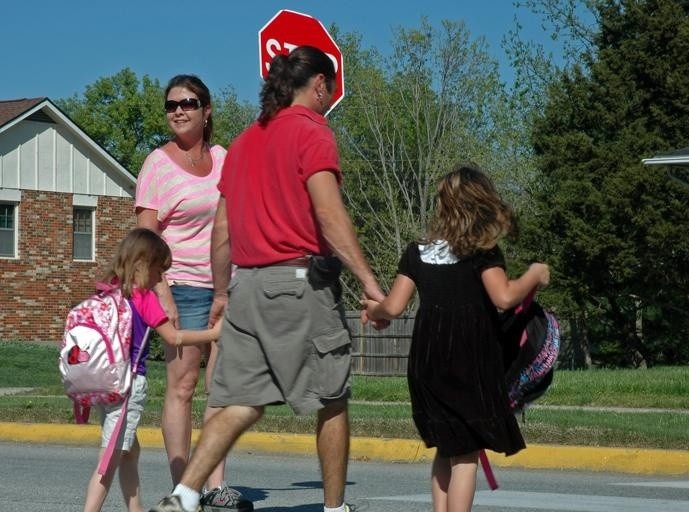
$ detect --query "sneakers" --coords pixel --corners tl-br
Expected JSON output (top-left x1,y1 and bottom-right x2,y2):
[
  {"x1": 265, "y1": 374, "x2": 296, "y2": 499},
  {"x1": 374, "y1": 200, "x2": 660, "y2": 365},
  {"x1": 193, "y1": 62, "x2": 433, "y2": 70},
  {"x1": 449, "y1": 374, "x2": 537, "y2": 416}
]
[
  {"x1": 150, "y1": 495, "x2": 204, "y2": 512},
  {"x1": 201, "y1": 480, "x2": 254, "y2": 512}
]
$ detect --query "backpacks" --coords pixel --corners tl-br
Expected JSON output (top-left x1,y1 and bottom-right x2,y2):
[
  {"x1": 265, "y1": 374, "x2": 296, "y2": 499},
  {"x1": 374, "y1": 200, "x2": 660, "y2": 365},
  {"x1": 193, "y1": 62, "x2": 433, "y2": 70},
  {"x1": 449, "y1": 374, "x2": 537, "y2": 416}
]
[
  {"x1": 58, "y1": 283, "x2": 154, "y2": 475},
  {"x1": 479, "y1": 289, "x2": 559, "y2": 492}
]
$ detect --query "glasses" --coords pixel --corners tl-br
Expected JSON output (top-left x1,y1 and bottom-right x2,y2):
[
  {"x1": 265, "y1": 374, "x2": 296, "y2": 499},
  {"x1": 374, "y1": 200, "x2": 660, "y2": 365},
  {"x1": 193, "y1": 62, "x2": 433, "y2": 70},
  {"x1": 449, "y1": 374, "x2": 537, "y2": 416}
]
[{"x1": 164, "y1": 98, "x2": 201, "y2": 113}]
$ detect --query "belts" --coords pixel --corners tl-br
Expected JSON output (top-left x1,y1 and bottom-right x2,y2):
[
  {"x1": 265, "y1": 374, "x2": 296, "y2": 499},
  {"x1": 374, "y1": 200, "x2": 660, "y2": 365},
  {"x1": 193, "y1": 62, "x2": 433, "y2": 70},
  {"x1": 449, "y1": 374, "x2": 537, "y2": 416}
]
[{"x1": 272, "y1": 257, "x2": 307, "y2": 266}]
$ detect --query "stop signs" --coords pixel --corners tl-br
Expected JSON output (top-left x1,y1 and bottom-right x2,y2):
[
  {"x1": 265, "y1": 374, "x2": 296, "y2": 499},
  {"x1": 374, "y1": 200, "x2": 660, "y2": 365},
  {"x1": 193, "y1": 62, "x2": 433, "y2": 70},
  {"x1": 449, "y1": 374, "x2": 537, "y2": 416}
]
[{"x1": 256, "y1": 7, "x2": 345, "y2": 119}]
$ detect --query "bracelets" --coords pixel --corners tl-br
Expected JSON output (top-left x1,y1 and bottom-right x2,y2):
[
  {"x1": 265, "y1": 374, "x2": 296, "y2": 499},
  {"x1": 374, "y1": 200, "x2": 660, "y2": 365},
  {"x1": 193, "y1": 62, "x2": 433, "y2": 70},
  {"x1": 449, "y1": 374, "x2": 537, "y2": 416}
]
[{"x1": 214, "y1": 293, "x2": 228, "y2": 296}]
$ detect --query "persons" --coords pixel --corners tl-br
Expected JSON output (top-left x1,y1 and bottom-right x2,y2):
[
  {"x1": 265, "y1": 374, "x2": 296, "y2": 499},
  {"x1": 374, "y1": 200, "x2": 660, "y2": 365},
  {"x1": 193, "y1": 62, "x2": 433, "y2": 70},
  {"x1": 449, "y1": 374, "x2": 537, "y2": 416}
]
[
  {"x1": 148, "y1": 44, "x2": 391, "y2": 512},
  {"x1": 359, "y1": 165, "x2": 551, "y2": 512},
  {"x1": 83, "y1": 227, "x2": 223, "y2": 511},
  {"x1": 132, "y1": 75, "x2": 254, "y2": 511}
]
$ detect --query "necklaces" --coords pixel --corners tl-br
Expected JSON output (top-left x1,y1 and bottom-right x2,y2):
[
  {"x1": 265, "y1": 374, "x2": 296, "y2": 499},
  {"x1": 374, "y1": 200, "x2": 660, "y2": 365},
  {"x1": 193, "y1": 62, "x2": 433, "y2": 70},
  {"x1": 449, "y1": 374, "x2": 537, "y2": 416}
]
[{"x1": 175, "y1": 143, "x2": 204, "y2": 168}]
